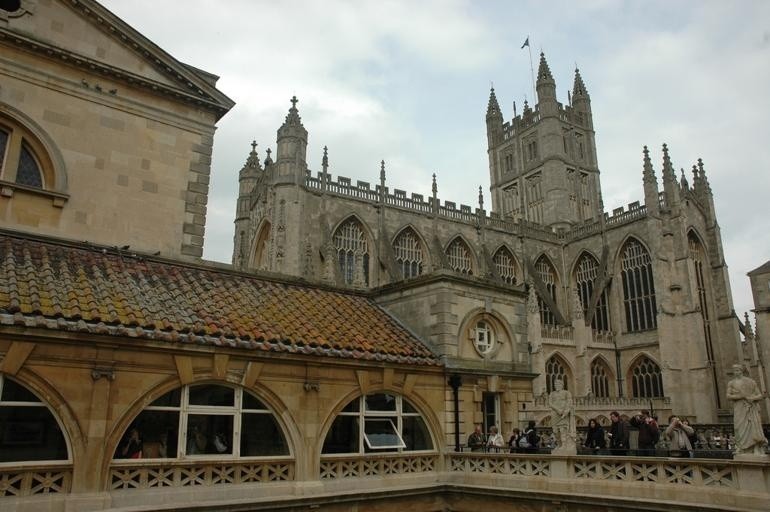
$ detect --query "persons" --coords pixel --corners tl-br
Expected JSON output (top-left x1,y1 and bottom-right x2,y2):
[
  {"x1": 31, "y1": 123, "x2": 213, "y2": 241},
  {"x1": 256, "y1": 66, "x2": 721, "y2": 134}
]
[
  {"x1": 681, "y1": 419, "x2": 698, "y2": 457},
  {"x1": 609, "y1": 412, "x2": 632, "y2": 455},
  {"x1": 710, "y1": 430, "x2": 736, "y2": 450},
  {"x1": 486, "y1": 424, "x2": 506, "y2": 453},
  {"x1": 508, "y1": 427, "x2": 520, "y2": 453},
  {"x1": 545, "y1": 378, "x2": 573, "y2": 448},
  {"x1": 519, "y1": 419, "x2": 540, "y2": 453},
  {"x1": 629, "y1": 409, "x2": 659, "y2": 459},
  {"x1": 725, "y1": 363, "x2": 765, "y2": 456},
  {"x1": 467, "y1": 426, "x2": 487, "y2": 452},
  {"x1": 664, "y1": 414, "x2": 699, "y2": 458},
  {"x1": 116, "y1": 428, "x2": 169, "y2": 459},
  {"x1": 582, "y1": 417, "x2": 606, "y2": 449}
]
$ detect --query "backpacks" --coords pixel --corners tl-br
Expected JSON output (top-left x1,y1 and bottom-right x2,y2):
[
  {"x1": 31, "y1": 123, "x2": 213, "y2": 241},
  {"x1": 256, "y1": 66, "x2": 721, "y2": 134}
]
[{"x1": 519, "y1": 429, "x2": 534, "y2": 448}]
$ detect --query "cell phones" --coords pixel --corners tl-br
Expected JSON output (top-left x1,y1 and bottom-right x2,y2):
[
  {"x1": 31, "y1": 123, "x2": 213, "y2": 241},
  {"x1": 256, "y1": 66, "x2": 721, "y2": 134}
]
[{"x1": 676, "y1": 417, "x2": 680, "y2": 421}]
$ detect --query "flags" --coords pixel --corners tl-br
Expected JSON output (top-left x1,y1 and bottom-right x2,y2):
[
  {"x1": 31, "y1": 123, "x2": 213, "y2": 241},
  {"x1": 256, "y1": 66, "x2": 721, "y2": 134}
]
[{"x1": 520, "y1": 40, "x2": 528, "y2": 52}]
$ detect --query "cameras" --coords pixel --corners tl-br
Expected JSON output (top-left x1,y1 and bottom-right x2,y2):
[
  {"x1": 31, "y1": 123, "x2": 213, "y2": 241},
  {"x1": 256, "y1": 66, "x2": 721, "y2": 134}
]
[{"x1": 639, "y1": 415, "x2": 646, "y2": 420}]
